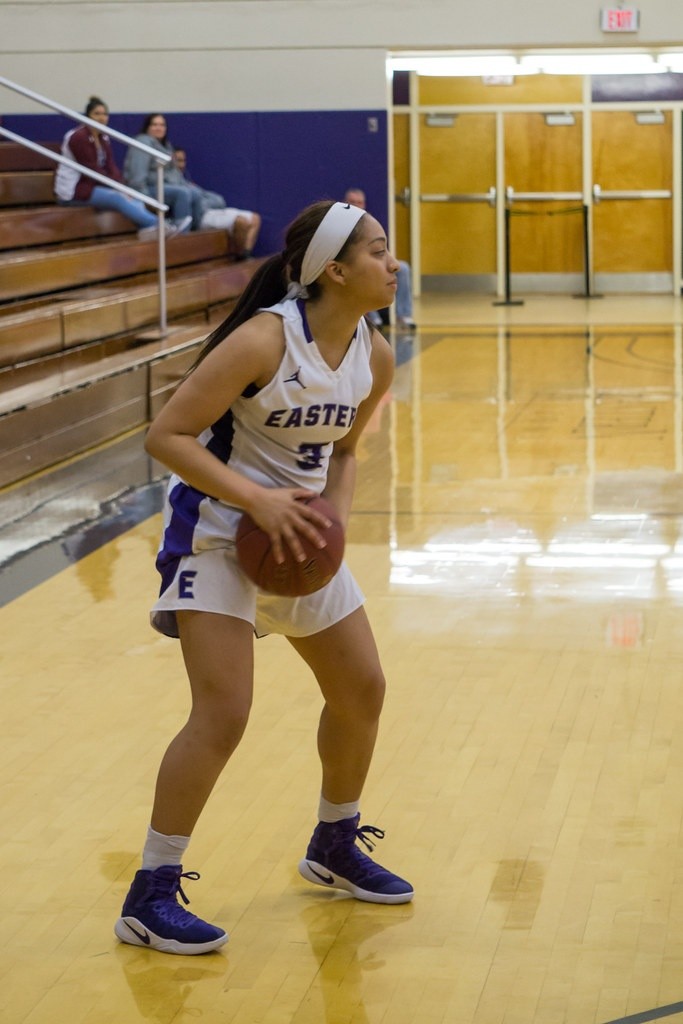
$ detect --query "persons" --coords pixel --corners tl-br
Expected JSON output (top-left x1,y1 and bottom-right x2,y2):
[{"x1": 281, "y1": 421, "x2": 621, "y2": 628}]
[
  {"x1": 173, "y1": 149, "x2": 261, "y2": 260},
  {"x1": 343, "y1": 188, "x2": 416, "y2": 332},
  {"x1": 122, "y1": 112, "x2": 204, "y2": 233},
  {"x1": 113, "y1": 203, "x2": 413, "y2": 955},
  {"x1": 54, "y1": 95, "x2": 193, "y2": 243}
]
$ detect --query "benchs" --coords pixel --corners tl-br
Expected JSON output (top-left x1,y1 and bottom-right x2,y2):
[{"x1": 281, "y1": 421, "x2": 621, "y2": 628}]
[{"x1": 0, "y1": 139, "x2": 285, "y2": 484}]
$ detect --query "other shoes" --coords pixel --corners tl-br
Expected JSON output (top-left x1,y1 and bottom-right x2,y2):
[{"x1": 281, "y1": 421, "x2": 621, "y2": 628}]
[
  {"x1": 166, "y1": 216, "x2": 192, "y2": 240},
  {"x1": 138, "y1": 223, "x2": 177, "y2": 240}
]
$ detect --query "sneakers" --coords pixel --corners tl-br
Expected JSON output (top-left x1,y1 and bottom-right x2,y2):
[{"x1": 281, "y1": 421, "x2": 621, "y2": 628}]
[
  {"x1": 114, "y1": 864, "x2": 228, "y2": 955},
  {"x1": 299, "y1": 812, "x2": 413, "y2": 904}
]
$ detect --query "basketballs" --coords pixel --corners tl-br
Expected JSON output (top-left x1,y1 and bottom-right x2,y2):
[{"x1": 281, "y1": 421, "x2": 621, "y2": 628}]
[{"x1": 234, "y1": 497, "x2": 347, "y2": 598}]
